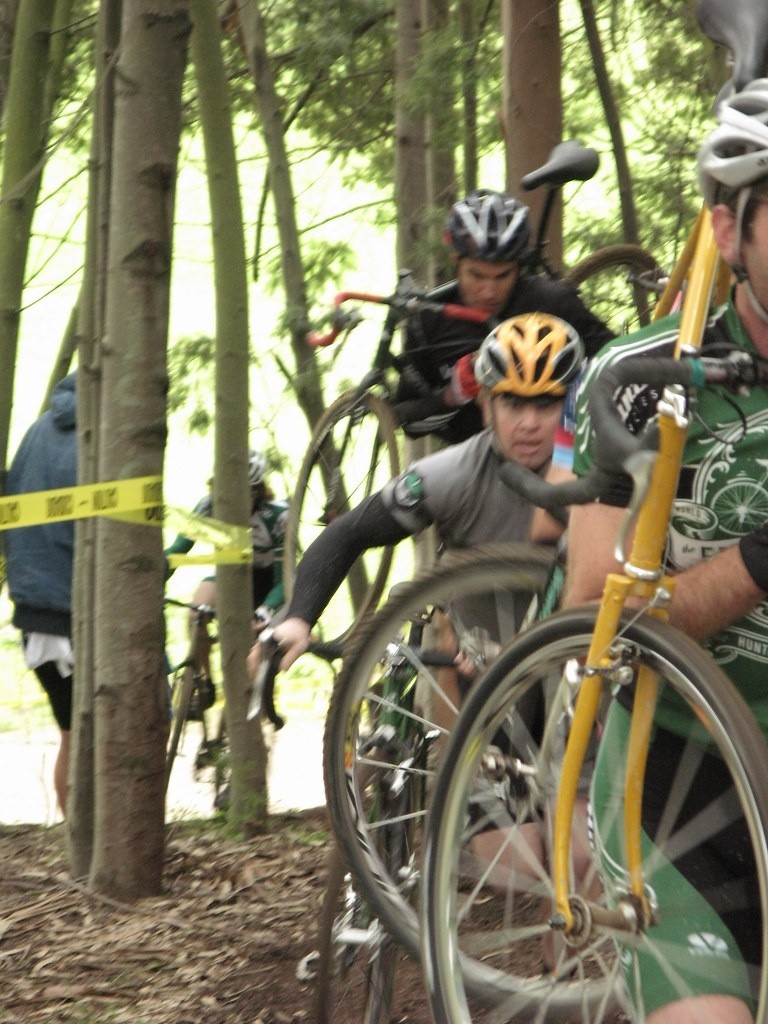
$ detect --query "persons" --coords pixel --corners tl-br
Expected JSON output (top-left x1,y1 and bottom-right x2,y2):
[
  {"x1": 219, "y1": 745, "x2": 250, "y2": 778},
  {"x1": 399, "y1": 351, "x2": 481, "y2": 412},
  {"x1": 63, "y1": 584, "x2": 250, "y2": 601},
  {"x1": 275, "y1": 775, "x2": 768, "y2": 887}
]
[
  {"x1": 162, "y1": 450, "x2": 292, "y2": 721},
  {"x1": 2, "y1": 367, "x2": 78, "y2": 822},
  {"x1": 245, "y1": 80, "x2": 768, "y2": 1024}
]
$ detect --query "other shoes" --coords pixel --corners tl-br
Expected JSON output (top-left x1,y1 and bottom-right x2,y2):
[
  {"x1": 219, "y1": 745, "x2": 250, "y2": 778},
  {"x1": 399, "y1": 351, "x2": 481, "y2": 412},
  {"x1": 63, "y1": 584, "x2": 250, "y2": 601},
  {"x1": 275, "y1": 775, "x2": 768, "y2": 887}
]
[
  {"x1": 188, "y1": 681, "x2": 215, "y2": 718},
  {"x1": 213, "y1": 784, "x2": 232, "y2": 811}
]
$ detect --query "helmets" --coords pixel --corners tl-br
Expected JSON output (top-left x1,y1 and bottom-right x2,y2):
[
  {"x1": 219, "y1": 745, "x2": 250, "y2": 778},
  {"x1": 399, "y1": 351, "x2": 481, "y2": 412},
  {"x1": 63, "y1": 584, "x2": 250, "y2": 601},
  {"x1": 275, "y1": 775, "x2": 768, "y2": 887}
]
[
  {"x1": 247, "y1": 449, "x2": 266, "y2": 487},
  {"x1": 693, "y1": 78, "x2": 768, "y2": 212},
  {"x1": 441, "y1": 189, "x2": 530, "y2": 263},
  {"x1": 475, "y1": 311, "x2": 585, "y2": 398}
]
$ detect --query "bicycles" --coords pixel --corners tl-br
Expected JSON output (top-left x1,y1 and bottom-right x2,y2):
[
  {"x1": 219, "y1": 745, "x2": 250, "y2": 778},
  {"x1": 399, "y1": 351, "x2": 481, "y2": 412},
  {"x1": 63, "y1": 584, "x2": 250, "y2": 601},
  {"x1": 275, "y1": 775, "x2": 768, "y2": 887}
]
[
  {"x1": 165, "y1": 595, "x2": 275, "y2": 802},
  {"x1": 278, "y1": 141, "x2": 683, "y2": 669},
  {"x1": 246, "y1": 200, "x2": 766, "y2": 1023}
]
